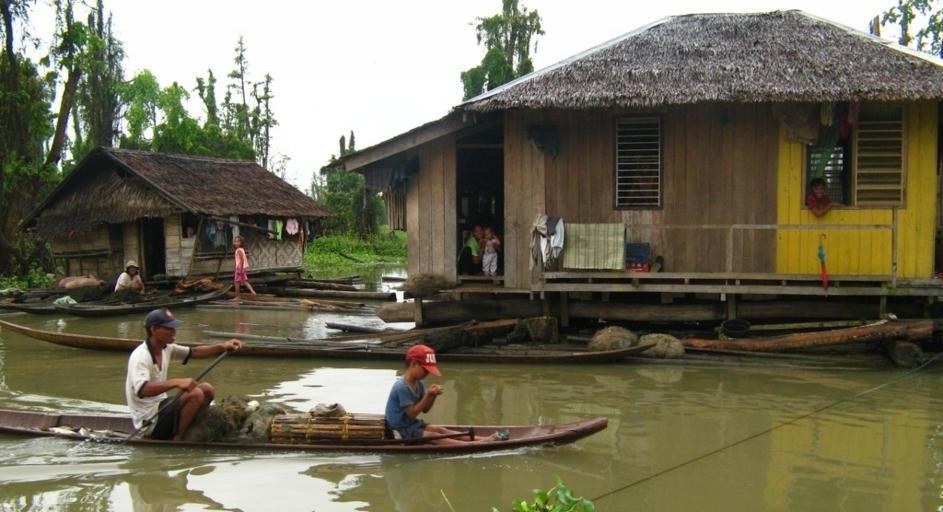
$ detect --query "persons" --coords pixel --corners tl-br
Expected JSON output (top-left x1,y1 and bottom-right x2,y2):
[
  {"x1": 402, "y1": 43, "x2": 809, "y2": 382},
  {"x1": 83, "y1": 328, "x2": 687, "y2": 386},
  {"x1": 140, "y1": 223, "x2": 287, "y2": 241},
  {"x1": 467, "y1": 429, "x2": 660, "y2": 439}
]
[
  {"x1": 479, "y1": 227, "x2": 502, "y2": 276},
  {"x1": 386, "y1": 344, "x2": 499, "y2": 446},
  {"x1": 458, "y1": 224, "x2": 484, "y2": 275},
  {"x1": 806, "y1": 179, "x2": 841, "y2": 218},
  {"x1": 229, "y1": 236, "x2": 256, "y2": 301},
  {"x1": 114, "y1": 260, "x2": 144, "y2": 295},
  {"x1": 125, "y1": 310, "x2": 241, "y2": 441}
]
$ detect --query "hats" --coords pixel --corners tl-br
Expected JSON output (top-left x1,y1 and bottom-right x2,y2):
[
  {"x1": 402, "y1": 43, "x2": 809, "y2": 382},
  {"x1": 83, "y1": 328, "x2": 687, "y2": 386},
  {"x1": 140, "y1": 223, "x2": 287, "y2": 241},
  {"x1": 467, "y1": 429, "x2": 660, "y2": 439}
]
[
  {"x1": 124, "y1": 260, "x2": 140, "y2": 272},
  {"x1": 143, "y1": 308, "x2": 185, "y2": 328},
  {"x1": 405, "y1": 344, "x2": 442, "y2": 377}
]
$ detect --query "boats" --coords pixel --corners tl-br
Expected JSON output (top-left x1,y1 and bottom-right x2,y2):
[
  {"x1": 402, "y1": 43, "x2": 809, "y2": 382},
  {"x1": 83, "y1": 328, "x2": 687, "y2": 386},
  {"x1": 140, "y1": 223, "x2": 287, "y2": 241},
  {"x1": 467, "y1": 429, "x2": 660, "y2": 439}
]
[
  {"x1": 52, "y1": 277, "x2": 234, "y2": 316},
  {"x1": 321, "y1": 321, "x2": 402, "y2": 337},
  {"x1": 0, "y1": 397, "x2": 610, "y2": 456},
  {"x1": 0, "y1": 290, "x2": 156, "y2": 316},
  {"x1": 235, "y1": 336, "x2": 658, "y2": 372},
  {"x1": 0, "y1": 318, "x2": 372, "y2": 369}
]
